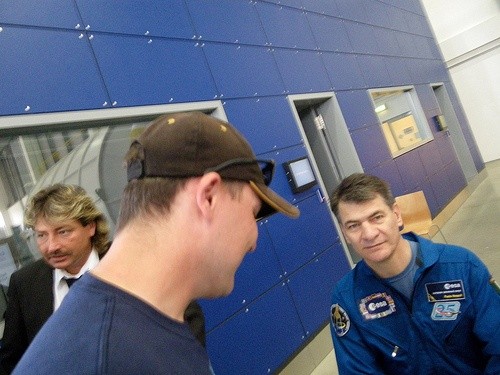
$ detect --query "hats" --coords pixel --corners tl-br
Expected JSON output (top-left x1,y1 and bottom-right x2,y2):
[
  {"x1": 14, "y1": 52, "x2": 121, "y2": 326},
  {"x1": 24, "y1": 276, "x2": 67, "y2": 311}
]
[{"x1": 126, "y1": 110, "x2": 302, "y2": 219}]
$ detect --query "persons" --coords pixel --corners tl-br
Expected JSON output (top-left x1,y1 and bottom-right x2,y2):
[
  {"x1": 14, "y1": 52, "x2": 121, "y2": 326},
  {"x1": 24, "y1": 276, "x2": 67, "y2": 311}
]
[
  {"x1": 0, "y1": 183, "x2": 113, "y2": 375},
  {"x1": 328, "y1": 172, "x2": 500, "y2": 375},
  {"x1": 8, "y1": 109, "x2": 301, "y2": 375}
]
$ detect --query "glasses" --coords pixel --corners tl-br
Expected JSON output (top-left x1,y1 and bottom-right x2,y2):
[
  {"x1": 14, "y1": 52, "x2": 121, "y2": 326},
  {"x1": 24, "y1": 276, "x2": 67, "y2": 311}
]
[{"x1": 205, "y1": 157, "x2": 275, "y2": 187}]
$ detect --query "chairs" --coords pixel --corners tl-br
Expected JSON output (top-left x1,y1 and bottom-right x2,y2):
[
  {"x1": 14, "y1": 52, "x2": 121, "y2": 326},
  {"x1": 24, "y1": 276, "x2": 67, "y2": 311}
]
[{"x1": 395, "y1": 190, "x2": 448, "y2": 244}]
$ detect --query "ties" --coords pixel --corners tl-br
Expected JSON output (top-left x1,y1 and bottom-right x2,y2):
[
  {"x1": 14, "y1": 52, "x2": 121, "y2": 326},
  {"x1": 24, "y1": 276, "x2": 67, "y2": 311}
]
[{"x1": 63, "y1": 276, "x2": 82, "y2": 289}]
